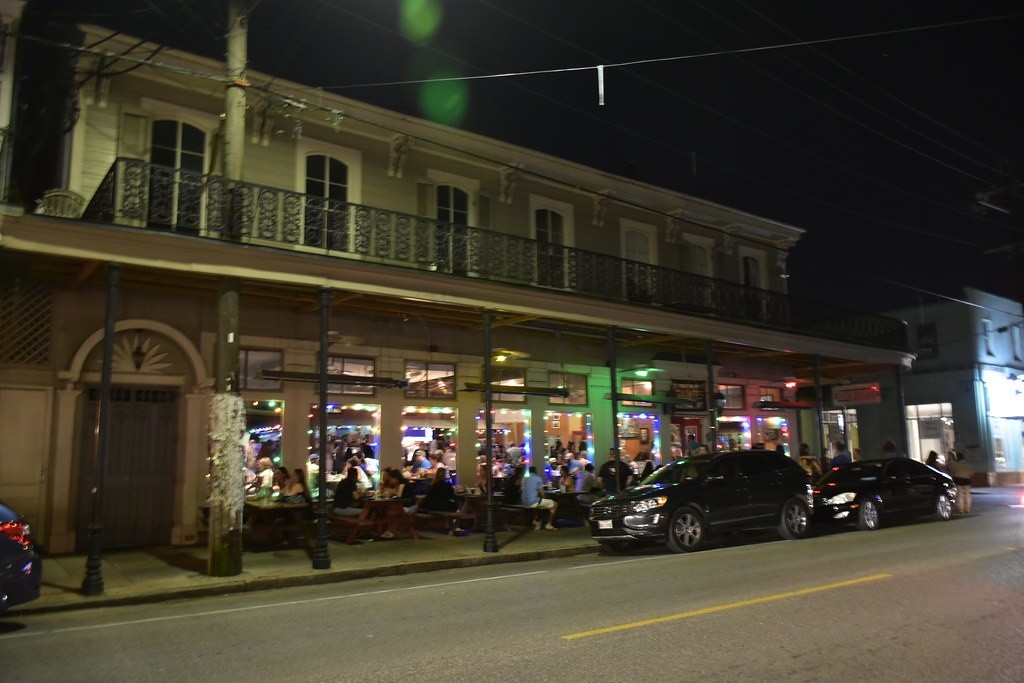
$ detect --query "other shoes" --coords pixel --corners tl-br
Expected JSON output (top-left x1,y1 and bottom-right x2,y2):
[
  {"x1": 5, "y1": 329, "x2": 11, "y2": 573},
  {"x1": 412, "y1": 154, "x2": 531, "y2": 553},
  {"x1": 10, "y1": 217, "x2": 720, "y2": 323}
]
[
  {"x1": 360, "y1": 535, "x2": 374, "y2": 541},
  {"x1": 533, "y1": 520, "x2": 543, "y2": 528},
  {"x1": 380, "y1": 530, "x2": 394, "y2": 538},
  {"x1": 545, "y1": 523, "x2": 557, "y2": 530}
]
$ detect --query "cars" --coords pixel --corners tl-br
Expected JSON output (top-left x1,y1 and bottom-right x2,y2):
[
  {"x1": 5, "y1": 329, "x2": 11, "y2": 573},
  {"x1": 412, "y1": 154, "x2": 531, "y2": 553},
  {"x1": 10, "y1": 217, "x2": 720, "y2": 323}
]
[
  {"x1": 0, "y1": 501, "x2": 43, "y2": 611},
  {"x1": 812, "y1": 456, "x2": 958, "y2": 531}
]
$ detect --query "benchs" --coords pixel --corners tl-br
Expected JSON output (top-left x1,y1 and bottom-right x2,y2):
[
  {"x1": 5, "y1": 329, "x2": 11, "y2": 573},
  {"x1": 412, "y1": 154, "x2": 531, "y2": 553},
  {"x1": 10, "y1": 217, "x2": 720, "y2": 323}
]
[{"x1": 244, "y1": 486, "x2": 590, "y2": 550}]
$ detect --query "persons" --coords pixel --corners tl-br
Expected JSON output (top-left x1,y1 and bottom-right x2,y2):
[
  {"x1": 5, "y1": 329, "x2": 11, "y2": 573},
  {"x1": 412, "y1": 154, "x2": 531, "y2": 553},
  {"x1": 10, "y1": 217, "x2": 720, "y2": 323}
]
[
  {"x1": 245, "y1": 430, "x2": 665, "y2": 540},
  {"x1": 671, "y1": 433, "x2": 973, "y2": 513}
]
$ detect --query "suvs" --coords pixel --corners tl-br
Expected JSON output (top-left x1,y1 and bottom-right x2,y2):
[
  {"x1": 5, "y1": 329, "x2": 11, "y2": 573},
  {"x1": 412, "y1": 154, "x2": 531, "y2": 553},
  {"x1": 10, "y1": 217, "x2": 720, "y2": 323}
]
[{"x1": 588, "y1": 450, "x2": 815, "y2": 553}]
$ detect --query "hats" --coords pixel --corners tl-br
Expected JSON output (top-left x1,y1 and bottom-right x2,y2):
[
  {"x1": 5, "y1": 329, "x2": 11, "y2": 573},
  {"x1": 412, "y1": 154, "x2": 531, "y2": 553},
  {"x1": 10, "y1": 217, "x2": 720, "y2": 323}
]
[
  {"x1": 415, "y1": 450, "x2": 426, "y2": 456},
  {"x1": 310, "y1": 454, "x2": 320, "y2": 460}
]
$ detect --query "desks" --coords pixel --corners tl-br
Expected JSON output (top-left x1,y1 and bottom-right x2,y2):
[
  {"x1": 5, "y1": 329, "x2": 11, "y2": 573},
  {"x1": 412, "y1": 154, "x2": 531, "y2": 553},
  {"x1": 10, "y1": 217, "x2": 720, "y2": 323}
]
[
  {"x1": 245, "y1": 497, "x2": 317, "y2": 550},
  {"x1": 448, "y1": 492, "x2": 512, "y2": 536},
  {"x1": 538, "y1": 490, "x2": 590, "y2": 528},
  {"x1": 348, "y1": 497, "x2": 419, "y2": 545}
]
